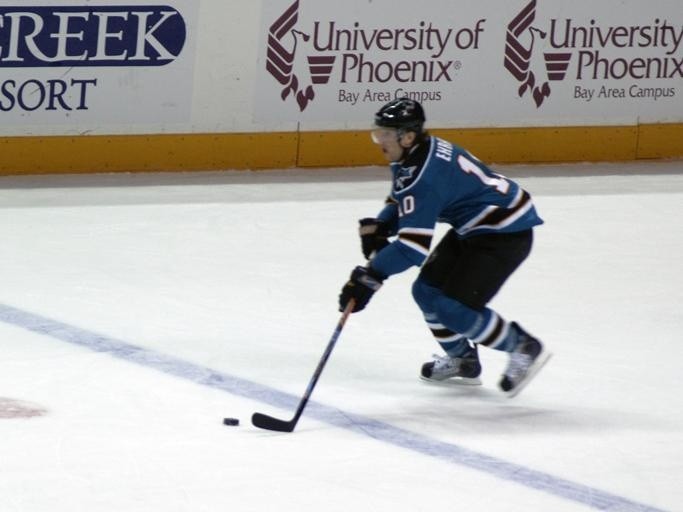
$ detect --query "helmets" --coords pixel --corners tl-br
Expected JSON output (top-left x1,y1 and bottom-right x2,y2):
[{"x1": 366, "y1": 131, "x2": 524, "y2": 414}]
[{"x1": 370, "y1": 96, "x2": 426, "y2": 146}]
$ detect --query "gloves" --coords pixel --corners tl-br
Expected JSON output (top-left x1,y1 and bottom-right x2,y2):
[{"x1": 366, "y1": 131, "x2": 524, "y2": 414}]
[
  {"x1": 357, "y1": 217, "x2": 391, "y2": 261},
  {"x1": 337, "y1": 264, "x2": 385, "y2": 313}
]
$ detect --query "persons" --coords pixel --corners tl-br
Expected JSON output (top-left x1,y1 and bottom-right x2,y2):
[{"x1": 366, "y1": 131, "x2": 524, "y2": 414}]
[{"x1": 334, "y1": 95, "x2": 544, "y2": 393}]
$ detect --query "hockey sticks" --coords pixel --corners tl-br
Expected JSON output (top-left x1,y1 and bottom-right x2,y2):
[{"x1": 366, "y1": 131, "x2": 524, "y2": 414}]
[{"x1": 252, "y1": 251, "x2": 376, "y2": 432}]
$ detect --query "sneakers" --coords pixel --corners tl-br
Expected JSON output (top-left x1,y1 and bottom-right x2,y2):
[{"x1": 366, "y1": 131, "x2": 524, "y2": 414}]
[
  {"x1": 421, "y1": 350, "x2": 482, "y2": 382},
  {"x1": 499, "y1": 335, "x2": 542, "y2": 391}
]
